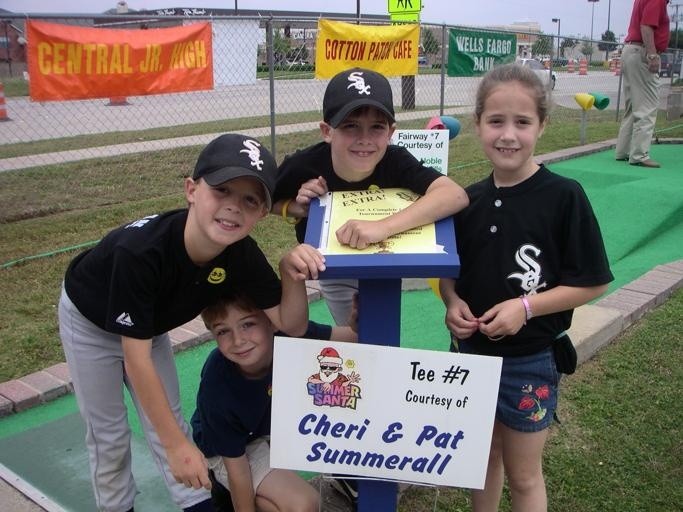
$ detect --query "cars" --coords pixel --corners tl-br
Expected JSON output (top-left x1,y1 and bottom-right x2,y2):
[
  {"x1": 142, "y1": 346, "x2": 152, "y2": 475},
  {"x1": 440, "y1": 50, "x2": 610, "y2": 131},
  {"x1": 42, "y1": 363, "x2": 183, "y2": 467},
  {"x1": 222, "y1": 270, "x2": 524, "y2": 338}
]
[
  {"x1": 552, "y1": 55, "x2": 568, "y2": 67},
  {"x1": 419, "y1": 46, "x2": 426, "y2": 65},
  {"x1": 514, "y1": 58, "x2": 557, "y2": 91},
  {"x1": 432, "y1": 45, "x2": 449, "y2": 68},
  {"x1": 277, "y1": 57, "x2": 306, "y2": 67}
]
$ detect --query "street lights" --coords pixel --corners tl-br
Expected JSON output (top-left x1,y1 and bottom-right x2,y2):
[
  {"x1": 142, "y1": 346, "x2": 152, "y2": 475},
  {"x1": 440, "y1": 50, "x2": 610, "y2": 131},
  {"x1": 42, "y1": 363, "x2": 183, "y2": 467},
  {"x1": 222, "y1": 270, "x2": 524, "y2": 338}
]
[{"x1": 550, "y1": 18, "x2": 560, "y2": 60}]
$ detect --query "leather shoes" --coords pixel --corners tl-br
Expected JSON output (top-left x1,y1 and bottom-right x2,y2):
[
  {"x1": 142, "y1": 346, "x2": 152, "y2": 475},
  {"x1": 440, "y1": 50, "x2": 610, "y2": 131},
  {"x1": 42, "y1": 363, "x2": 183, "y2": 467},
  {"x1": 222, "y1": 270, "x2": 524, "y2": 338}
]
[{"x1": 630, "y1": 157, "x2": 660, "y2": 167}]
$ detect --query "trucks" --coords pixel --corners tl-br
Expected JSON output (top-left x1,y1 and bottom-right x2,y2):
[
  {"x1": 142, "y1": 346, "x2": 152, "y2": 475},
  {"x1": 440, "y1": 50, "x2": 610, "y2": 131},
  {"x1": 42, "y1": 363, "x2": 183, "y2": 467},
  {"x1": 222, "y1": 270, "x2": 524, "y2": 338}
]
[{"x1": 658, "y1": 47, "x2": 682, "y2": 77}]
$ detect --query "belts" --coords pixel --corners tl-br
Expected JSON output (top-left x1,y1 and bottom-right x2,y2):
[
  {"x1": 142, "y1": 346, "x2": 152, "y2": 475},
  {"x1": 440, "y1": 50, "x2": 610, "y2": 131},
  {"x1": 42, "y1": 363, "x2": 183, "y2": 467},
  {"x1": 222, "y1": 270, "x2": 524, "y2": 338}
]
[{"x1": 626, "y1": 41, "x2": 643, "y2": 48}]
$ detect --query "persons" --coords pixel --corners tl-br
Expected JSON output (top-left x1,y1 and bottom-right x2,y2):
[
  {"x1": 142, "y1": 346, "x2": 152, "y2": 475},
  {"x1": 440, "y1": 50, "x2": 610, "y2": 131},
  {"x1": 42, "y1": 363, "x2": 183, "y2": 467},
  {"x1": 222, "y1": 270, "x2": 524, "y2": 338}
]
[
  {"x1": 56, "y1": 134, "x2": 326, "y2": 511},
  {"x1": 268, "y1": 68, "x2": 472, "y2": 328},
  {"x1": 612, "y1": 1, "x2": 672, "y2": 167},
  {"x1": 435, "y1": 63, "x2": 617, "y2": 512},
  {"x1": 190, "y1": 289, "x2": 362, "y2": 511}
]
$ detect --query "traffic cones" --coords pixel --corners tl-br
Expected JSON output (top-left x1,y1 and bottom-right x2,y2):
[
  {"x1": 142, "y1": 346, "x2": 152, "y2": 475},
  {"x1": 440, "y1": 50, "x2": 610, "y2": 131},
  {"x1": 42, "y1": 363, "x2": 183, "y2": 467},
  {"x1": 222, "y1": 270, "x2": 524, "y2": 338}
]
[
  {"x1": 610, "y1": 60, "x2": 615, "y2": 72},
  {"x1": 1, "y1": 81, "x2": 14, "y2": 122},
  {"x1": 567, "y1": 61, "x2": 574, "y2": 74},
  {"x1": 615, "y1": 60, "x2": 620, "y2": 76},
  {"x1": 578, "y1": 59, "x2": 587, "y2": 74},
  {"x1": 103, "y1": 96, "x2": 133, "y2": 107}
]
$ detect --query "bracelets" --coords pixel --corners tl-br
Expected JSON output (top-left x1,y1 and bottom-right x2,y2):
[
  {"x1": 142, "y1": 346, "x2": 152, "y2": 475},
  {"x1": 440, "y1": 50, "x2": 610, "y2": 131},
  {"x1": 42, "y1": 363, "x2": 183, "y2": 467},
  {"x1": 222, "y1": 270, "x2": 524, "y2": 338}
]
[
  {"x1": 520, "y1": 294, "x2": 535, "y2": 321},
  {"x1": 282, "y1": 199, "x2": 302, "y2": 225},
  {"x1": 645, "y1": 54, "x2": 658, "y2": 60}
]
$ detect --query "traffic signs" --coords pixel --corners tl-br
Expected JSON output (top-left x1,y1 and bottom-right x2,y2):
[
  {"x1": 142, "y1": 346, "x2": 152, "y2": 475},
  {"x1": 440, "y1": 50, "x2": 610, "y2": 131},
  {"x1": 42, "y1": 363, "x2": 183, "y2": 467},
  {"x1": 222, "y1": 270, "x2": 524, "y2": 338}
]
[{"x1": 387, "y1": 0, "x2": 422, "y2": 27}]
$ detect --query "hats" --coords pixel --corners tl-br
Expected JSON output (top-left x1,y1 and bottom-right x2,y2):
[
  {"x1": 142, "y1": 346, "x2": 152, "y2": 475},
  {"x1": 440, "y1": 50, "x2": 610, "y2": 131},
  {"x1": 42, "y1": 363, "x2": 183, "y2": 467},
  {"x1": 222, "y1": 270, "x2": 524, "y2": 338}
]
[
  {"x1": 323, "y1": 68, "x2": 396, "y2": 129},
  {"x1": 193, "y1": 134, "x2": 279, "y2": 213}
]
